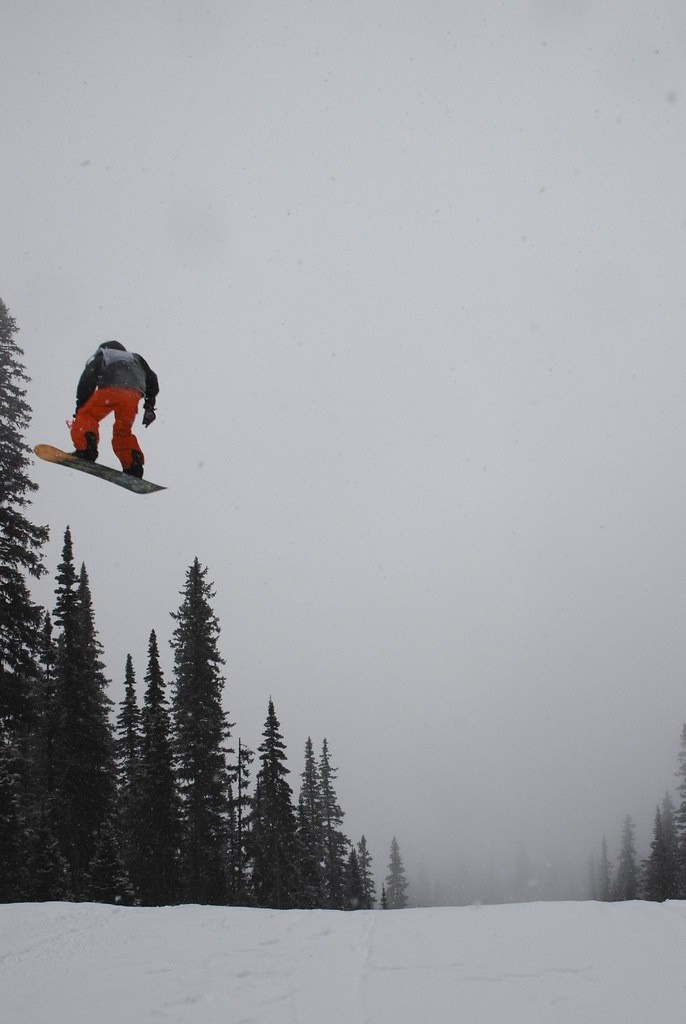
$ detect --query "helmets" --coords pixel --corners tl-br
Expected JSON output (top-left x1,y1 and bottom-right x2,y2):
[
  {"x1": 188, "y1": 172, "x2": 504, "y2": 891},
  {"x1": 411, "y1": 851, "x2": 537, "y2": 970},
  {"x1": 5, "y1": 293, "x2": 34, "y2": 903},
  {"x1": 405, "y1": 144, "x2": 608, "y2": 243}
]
[{"x1": 101, "y1": 338, "x2": 126, "y2": 351}]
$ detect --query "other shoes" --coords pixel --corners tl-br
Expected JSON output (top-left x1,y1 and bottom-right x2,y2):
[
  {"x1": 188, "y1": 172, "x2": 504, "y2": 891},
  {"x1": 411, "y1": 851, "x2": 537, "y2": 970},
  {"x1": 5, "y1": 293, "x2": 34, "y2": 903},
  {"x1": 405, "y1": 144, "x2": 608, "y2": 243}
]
[
  {"x1": 122, "y1": 465, "x2": 143, "y2": 478},
  {"x1": 69, "y1": 450, "x2": 98, "y2": 462}
]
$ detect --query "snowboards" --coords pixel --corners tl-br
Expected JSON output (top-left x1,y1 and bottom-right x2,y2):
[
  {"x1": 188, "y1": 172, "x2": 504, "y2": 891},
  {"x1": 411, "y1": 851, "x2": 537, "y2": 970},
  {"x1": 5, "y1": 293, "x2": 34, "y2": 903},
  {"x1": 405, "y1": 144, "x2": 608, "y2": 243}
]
[{"x1": 33, "y1": 443, "x2": 167, "y2": 494}]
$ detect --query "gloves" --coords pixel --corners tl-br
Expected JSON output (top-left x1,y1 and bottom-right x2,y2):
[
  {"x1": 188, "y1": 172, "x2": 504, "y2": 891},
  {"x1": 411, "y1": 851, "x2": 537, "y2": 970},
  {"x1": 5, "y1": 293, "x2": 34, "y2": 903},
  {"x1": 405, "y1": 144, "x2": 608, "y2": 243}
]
[
  {"x1": 73, "y1": 405, "x2": 84, "y2": 417},
  {"x1": 142, "y1": 408, "x2": 156, "y2": 428}
]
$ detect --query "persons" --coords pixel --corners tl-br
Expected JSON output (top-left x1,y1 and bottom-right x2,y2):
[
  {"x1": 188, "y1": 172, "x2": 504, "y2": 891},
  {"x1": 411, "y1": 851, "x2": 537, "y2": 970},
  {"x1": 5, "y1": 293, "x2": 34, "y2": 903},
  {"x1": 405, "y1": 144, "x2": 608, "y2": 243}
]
[{"x1": 68, "y1": 340, "x2": 160, "y2": 479}]
